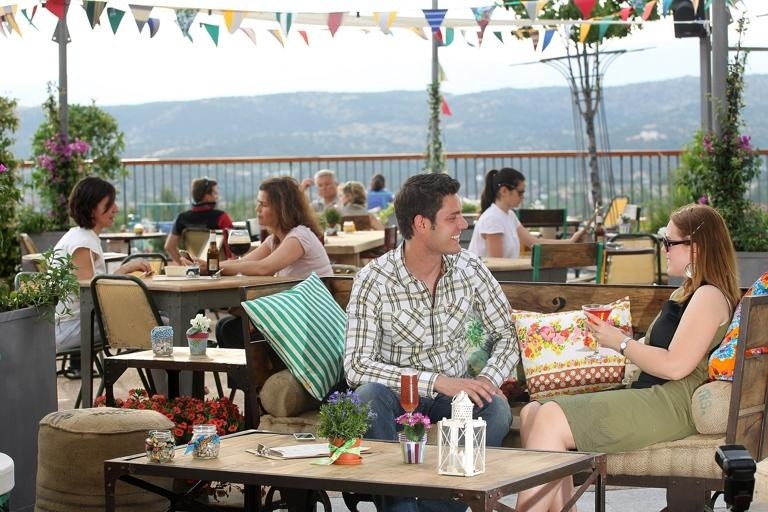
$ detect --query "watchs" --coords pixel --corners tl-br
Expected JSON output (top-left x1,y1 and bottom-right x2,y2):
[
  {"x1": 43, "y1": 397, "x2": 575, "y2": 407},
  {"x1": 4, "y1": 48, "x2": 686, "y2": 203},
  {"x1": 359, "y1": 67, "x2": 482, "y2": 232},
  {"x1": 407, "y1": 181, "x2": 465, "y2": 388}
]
[{"x1": 619, "y1": 338, "x2": 631, "y2": 356}]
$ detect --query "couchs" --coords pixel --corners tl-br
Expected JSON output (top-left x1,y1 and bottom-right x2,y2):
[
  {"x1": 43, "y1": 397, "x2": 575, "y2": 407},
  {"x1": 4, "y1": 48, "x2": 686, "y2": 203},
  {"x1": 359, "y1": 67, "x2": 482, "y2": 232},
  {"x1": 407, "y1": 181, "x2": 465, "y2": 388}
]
[{"x1": 237, "y1": 271, "x2": 767, "y2": 512}]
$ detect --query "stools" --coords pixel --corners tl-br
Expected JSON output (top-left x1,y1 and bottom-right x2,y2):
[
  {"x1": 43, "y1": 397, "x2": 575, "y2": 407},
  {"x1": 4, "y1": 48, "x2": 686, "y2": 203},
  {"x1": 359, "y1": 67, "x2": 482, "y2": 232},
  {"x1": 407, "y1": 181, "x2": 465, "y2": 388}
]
[
  {"x1": 29, "y1": 407, "x2": 175, "y2": 511},
  {"x1": 0, "y1": 448, "x2": 18, "y2": 512}
]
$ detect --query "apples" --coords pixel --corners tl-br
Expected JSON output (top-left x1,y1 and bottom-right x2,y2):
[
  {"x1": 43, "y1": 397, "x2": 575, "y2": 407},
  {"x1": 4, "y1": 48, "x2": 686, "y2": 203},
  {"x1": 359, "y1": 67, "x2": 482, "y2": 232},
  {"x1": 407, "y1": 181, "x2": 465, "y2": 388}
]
[{"x1": 133, "y1": 224, "x2": 144, "y2": 234}]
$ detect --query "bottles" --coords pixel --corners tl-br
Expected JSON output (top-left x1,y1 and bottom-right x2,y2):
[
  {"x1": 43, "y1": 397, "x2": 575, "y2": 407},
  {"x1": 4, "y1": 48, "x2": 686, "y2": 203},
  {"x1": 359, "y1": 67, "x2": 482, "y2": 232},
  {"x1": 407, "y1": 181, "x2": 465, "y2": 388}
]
[
  {"x1": 207, "y1": 229, "x2": 219, "y2": 276},
  {"x1": 145, "y1": 429, "x2": 175, "y2": 463},
  {"x1": 191, "y1": 424, "x2": 220, "y2": 459},
  {"x1": 151, "y1": 326, "x2": 173, "y2": 356},
  {"x1": 594, "y1": 213, "x2": 607, "y2": 249}
]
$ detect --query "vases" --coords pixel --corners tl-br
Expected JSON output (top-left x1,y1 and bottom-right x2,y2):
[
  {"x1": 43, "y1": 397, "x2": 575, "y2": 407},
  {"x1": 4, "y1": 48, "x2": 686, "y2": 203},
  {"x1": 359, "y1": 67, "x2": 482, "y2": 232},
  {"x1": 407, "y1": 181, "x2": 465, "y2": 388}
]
[
  {"x1": 330, "y1": 436, "x2": 365, "y2": 465},
  {"x1": 396, "y1": 432, "x2": 428, "y2": 463},
  {"x1": 185, "y1": 334, "x2": 211, "y2": 357}
]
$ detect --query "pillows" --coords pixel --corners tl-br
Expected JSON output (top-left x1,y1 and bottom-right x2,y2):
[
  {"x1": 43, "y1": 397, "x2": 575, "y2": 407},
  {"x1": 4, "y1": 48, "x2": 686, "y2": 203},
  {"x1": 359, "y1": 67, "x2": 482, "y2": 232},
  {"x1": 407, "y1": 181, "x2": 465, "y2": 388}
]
[
  {"x1": 461, "y1": 298, "x2": 543, "y2": 403},
  {"x1": 707, "y1": 273, "x2": 767, "y2": 384},
  {"x1": 687, "y1": 378, "x2": 735, "y2": 435},
  {"x1": 512, "y1": 294, "x2": 633, "y2": 408},
  {"x1": 240, "y1": 269, "x2": 350, "y2": 402}
]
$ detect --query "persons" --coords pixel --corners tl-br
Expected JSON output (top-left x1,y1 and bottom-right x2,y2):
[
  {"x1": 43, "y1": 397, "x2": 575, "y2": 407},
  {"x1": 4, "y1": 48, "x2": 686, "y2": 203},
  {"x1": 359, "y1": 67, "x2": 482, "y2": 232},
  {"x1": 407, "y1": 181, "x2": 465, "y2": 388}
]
[
  {"x1": 334, "y1": 181, "x2": 385, "y2": 230},
  {"x1": 45, "y1": 176, "x2": 152, "y2": 353},
  {"x1": 468, "y1": 168, "x2": 586, "y2": 260},
  {"x1": 514, "y1": 205, "x2": 741, "y2": 512},
  {"x1": 366, "y1": 174, "x2": 393, "y2": 209},
  {"x1": 342, "y1": 174, "x2": 521, "y2": 512},
  {"x1": 300, "y1": 169, "x2": 339, "y2": 213},
  {"x1": 180, "y1": 175, "x2": 334, "y2": 416},
  {"x1": 164, "y1": 177, "x2": 236, "y2": 267}
]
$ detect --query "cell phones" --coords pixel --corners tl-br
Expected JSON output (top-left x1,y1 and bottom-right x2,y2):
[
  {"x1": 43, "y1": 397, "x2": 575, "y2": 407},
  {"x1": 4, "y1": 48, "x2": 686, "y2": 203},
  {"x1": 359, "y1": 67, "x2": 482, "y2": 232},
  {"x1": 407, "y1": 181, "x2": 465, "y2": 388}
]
[{"x1": 293, "y1": 433, "x2": 315, "y2": 441}]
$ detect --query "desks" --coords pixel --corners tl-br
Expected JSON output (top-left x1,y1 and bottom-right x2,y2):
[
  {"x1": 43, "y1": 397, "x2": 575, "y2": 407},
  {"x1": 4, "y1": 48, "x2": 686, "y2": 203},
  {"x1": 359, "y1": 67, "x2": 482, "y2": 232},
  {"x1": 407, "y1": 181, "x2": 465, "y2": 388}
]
[
  {"x1": 100, "y1": 427, "x2": 607, "y2": 512},
  {"x1": 100, "y1": 346, "x2": 246, "y2": 429}
]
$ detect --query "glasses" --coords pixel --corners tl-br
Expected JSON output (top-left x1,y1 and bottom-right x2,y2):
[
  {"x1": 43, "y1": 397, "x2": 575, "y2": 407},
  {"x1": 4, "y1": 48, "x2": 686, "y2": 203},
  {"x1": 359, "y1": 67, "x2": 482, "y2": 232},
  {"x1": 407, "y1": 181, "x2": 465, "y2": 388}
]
[
  {"x1": 663, "y1": 232, "x2": 691, "y2": 253},
  {"x1": 499, "y1": 183, "x2": 525, "y2": 197}
]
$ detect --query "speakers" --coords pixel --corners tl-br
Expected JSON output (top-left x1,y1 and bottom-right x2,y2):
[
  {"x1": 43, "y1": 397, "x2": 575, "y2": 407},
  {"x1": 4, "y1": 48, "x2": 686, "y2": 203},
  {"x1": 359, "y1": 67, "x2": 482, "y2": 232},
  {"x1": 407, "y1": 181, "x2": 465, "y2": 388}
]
[{"x1": 672, "y1": 0, "x2": 707, "y2": 38}]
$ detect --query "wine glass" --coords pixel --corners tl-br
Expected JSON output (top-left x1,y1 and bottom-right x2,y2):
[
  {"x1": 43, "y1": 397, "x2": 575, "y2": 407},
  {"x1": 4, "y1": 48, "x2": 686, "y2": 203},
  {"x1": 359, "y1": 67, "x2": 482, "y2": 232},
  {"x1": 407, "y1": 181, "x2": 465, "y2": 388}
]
[
  {"x1": 227, "y1": 230, "x2": 251, "y2": 278},
  {"x1": 582, "y1": 304, "x2": 613, "y2": 361},
  {"x1": 400, "y1": 370, "x2": 419, "y2": 414}
]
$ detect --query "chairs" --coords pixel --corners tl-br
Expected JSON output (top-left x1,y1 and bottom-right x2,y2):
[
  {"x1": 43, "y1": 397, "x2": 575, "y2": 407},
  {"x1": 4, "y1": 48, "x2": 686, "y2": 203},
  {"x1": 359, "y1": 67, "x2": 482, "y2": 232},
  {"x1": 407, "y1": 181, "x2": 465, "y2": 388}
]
[
  {"x1": 601, "y1": 194, "x2": 636, "y2": 230},
  {"x1": 70, "y1": 273, "x2": 163, "y2": 416},
  {"x1": 18, "y1": 231, "x2": 37, "y2": 254}
]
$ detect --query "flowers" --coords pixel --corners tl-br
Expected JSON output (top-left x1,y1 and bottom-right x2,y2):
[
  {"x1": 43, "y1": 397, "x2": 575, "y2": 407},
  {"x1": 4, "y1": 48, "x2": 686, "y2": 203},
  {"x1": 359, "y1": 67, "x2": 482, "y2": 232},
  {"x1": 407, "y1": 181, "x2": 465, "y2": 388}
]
[
  {"x1": 86, "y1": 384, "x2": 244, "y2": 445},
  {"x1": 314, "y1": 387, "x2": 381, "y2": 439},
  {"x1": 396, "y1": 411, "x2": 432, "y2": 440},
  {"x1": 185, "y1": 313, "x2": 213, "y2": 337}
]
